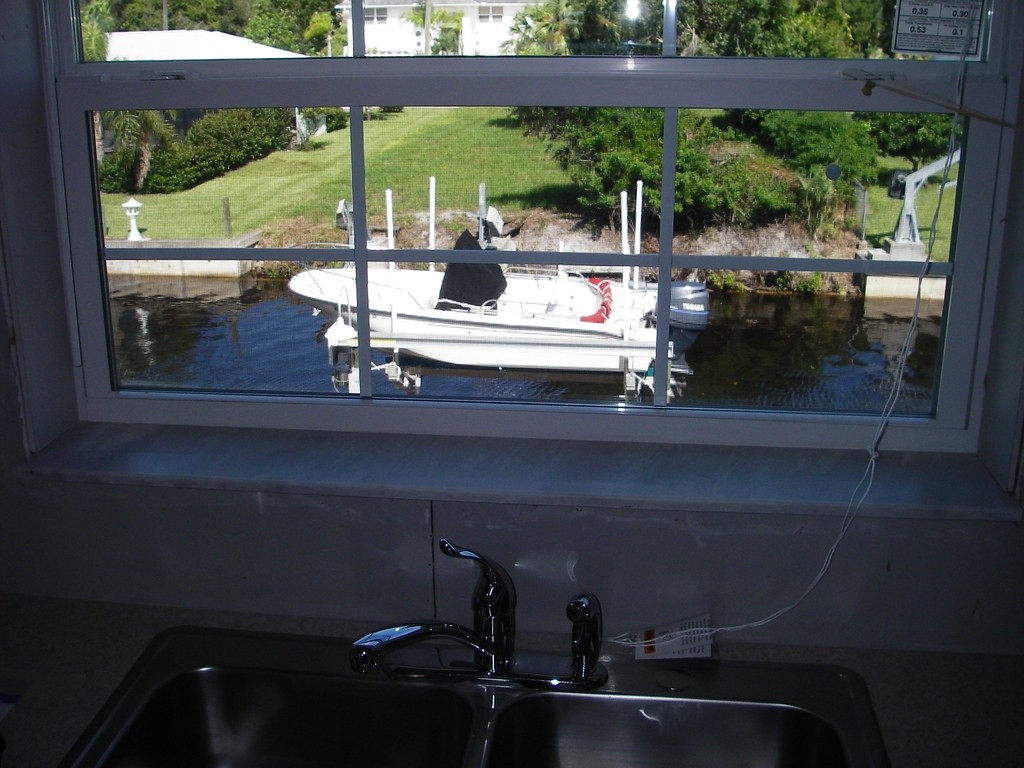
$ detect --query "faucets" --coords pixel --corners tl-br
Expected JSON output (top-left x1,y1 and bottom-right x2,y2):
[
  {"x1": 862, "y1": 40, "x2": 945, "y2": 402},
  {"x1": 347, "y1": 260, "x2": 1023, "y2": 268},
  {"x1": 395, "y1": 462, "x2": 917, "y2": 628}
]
[
  {"x1": 566, "y1": 591, "x2": 604, "y2": 670},
  {"x1": 348, "y1": 538, "x2": 518, "y2": 677}
]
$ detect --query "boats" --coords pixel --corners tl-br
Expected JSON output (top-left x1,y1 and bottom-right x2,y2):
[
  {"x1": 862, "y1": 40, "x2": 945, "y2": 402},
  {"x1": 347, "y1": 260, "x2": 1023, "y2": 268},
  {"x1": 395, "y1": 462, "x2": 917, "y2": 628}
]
[{"x1": 287, "y1": 173, "x2": 710, "y2": 399}]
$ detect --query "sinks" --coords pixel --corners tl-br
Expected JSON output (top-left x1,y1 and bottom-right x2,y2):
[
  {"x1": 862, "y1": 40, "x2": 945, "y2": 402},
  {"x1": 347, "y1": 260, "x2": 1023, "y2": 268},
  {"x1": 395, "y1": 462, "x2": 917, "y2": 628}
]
[
  {"x1": 56, "y1": 624, "x2": 486, "y2": 768},
  {"x1": 474, "y1": 654, "x2": 893, "y2": 768}
]
